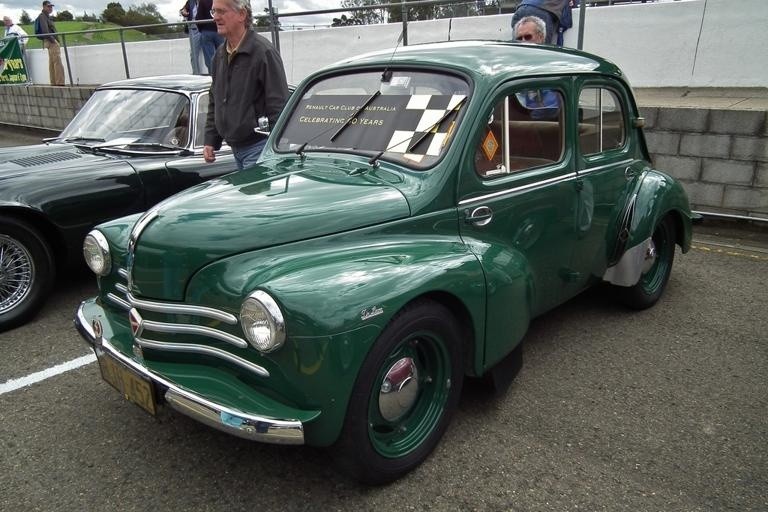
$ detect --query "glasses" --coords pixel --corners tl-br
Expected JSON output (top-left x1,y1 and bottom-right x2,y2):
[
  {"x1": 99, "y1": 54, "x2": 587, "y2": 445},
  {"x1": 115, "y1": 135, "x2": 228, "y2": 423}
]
[
  {"x1": 209, "y1": 9, "x2": 231, "y2": 18},
  {"x1": 515, "y1": 33, "x2": 531, "y2": 40}
]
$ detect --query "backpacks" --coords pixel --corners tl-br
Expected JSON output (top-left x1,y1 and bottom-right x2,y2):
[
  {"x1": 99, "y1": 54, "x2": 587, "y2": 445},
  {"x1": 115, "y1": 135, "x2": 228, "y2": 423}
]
[{"x1": 34, "y1": 13, "x2": 49, "y2": 39}]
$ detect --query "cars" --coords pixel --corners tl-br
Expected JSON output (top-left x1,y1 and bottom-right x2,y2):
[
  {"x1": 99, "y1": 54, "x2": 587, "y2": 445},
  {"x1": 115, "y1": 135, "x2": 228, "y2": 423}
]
[
  {"x1": 77, "y1": 41, "x2": 692, "y2": 484},
  {"x1": 0, "y1": 75, "x2": 301, "y2": 330}
]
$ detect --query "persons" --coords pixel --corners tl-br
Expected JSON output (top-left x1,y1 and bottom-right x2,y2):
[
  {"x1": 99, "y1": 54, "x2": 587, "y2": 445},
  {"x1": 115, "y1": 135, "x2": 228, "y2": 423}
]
[
  {"x1": 512, "y1": 16, "x2": 562, "y2": 108},
  {"x1": 202, "y1": 0, "x2": 291, "y2": 170},
  {"x1": 180, "y1": 1, "x2": 212, "y2": 75},
  {"x1": 39, "y1": 1, "x2": 65, "y2": 86},
  {"x1": 2, "y1": 16, "x2": 30, "y2": 54},
  {"x1": 512, "y1": 0, "x2": 579, "y2": 48},
  {"x1": 195, "y1": 1, "x2": 225, "y2": 75}
]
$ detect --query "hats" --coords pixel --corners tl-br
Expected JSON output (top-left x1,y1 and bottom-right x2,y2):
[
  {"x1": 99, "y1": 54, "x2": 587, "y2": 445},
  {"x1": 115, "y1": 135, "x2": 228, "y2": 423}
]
[{"x1": 44, "y1": 1, "x2": 56, "y2": 7}]
